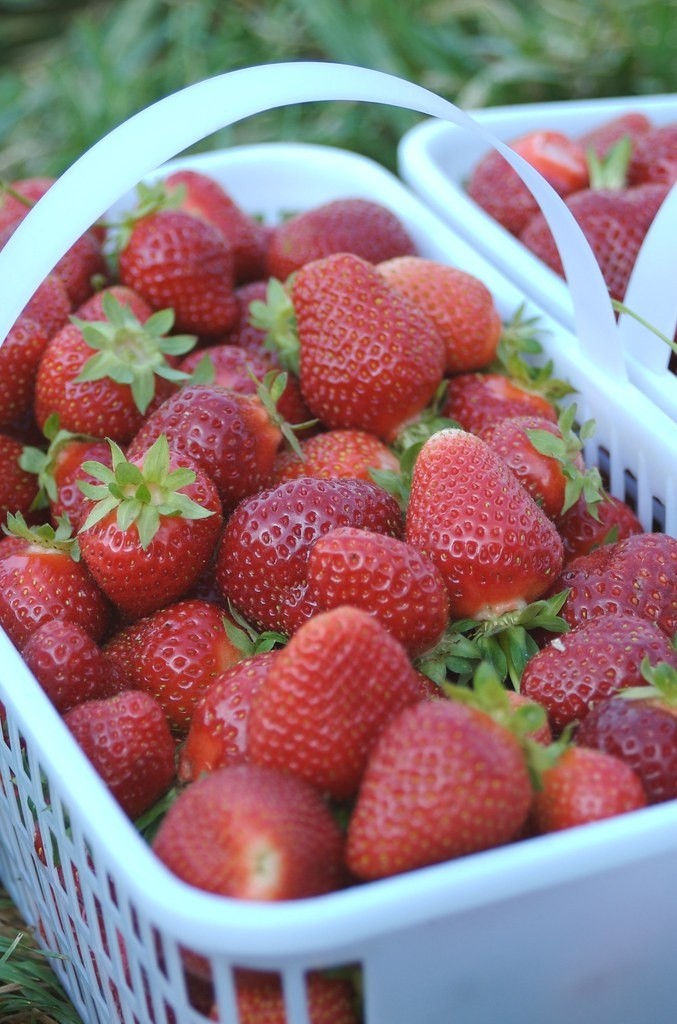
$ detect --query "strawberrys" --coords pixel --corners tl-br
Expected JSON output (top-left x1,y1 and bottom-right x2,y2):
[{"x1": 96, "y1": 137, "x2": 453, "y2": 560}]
[{"x1": 1, "y1": 116, "x2": 676, "y2": 1024}]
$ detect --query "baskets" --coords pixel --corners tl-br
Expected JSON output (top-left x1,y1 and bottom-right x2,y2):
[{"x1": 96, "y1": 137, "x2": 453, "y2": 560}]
[
  {"x1": 397, "y1": 92, "x2": 677, "y2": 412},
  {"x1": 0, "y1": 62, "x2": 677, "y2": 1024}
]
